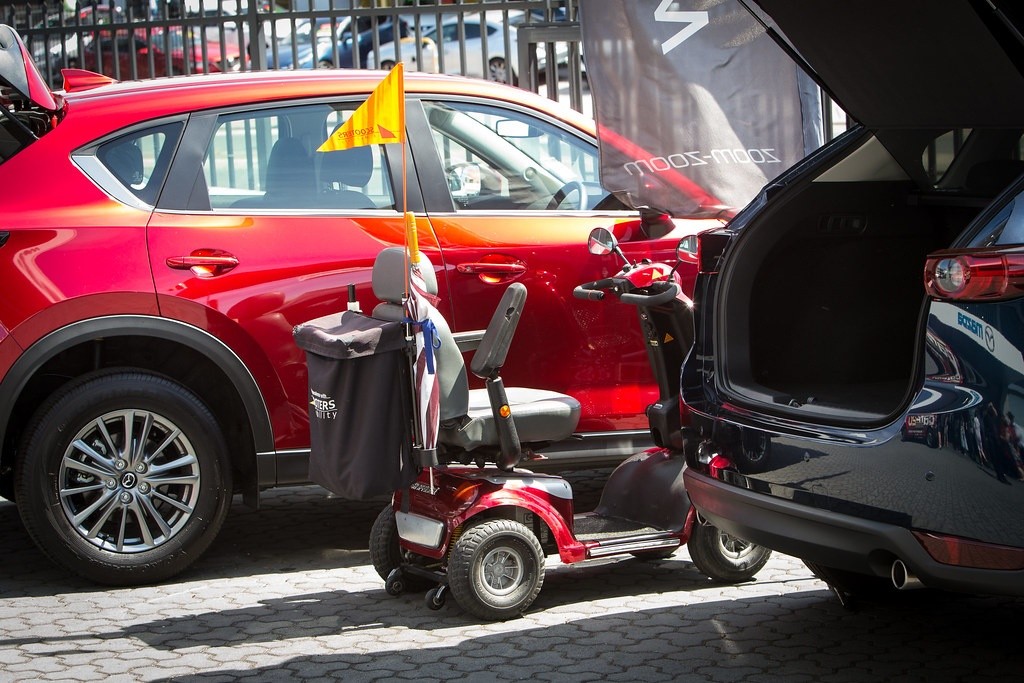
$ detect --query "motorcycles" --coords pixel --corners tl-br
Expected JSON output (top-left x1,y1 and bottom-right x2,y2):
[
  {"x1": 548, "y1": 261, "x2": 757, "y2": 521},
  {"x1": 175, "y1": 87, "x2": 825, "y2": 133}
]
[{"x1": 368, "y1": 223, "x2": 773, "y2": 621}]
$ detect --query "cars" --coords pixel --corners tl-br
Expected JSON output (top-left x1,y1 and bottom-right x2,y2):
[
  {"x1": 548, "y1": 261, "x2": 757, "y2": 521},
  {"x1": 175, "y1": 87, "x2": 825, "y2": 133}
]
[
  {"x1": 369, "y1": 7, "x2": 569, "y2": 89},
  {"x1": 76, "y1": 24, "x2": 249, "y2": 78},
  {"x1": 0, "y1": 20, "x2": 736, "y2": 587},
  {"x1": 672, "y1": 2, "x2": 1024, "y2": 657},
  {"x1": 240, "y1": 7, "x2": 405, "y2": 81},
  {"x1": 16, "y1": 12, "x2": 92, "y2": 85},
  {"x1": 570, "y1": 35, "x2": 659, "y2": 86}
]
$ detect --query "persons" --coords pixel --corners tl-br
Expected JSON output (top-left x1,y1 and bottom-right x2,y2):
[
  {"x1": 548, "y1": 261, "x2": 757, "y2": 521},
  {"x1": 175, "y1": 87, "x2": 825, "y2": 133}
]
[{"x1": 935, "y1": 406, "x2": 1024, "y2": 487}]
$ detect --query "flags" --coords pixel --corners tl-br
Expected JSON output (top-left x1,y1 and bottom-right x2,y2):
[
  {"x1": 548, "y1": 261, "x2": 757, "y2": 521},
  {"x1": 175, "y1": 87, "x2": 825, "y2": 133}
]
[{"x1": 579, "y1": 0, "x2": 822, "y2": 223}]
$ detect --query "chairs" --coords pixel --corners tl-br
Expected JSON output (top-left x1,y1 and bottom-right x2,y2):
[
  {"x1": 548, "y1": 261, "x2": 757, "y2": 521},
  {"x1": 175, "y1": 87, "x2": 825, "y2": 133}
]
[
  {"x1": 372, "y1": 247, "x2": 581, "y2": 450},
  {"x1": 316, "y1": 122, "x2": 378, "y2": 208},
  {"x1": 264, "y1": 138, "x2": 317, "y2": 210}
]
